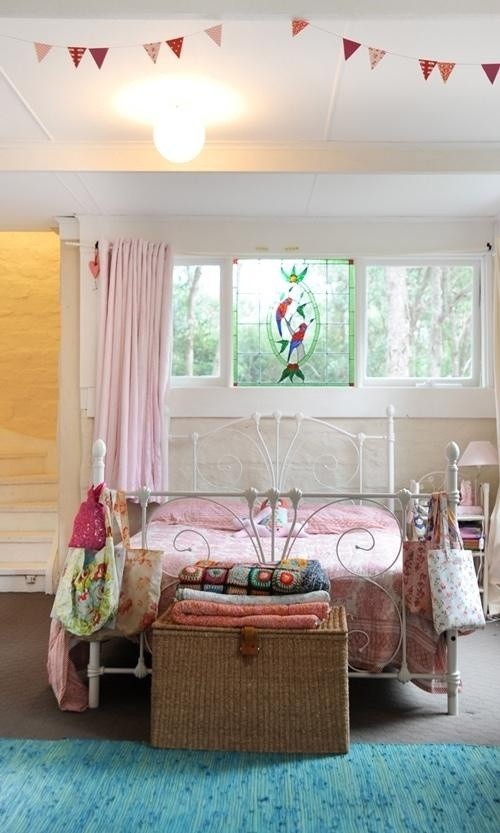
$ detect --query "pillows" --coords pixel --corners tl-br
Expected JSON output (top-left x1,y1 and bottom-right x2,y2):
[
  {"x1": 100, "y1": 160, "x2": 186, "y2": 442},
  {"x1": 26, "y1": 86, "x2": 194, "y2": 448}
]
[
  {"x1": 150, "y1": 499, "x2": 267, "y2": 531},
  {"x1": 258, "y1": 500, "x2": 396, "y2": 535}
]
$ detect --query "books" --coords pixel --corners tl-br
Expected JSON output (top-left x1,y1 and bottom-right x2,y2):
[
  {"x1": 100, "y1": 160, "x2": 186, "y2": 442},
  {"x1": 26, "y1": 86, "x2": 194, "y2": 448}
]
[{"x1": 460, "y1": 526, "x2": 484, "y2": 552}]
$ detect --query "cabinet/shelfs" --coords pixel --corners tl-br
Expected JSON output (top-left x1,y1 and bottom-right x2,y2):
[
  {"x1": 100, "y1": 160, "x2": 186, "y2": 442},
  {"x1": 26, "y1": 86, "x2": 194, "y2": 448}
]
[{"x1": 412, "y1": 470, "x2": 490, "y2": 621}]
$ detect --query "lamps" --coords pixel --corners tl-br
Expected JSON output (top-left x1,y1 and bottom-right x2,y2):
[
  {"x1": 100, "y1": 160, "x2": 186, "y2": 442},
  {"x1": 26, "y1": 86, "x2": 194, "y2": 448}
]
[
  {"x1": 455, "y1": 438, "x2": 500, "y2": 505},
  {"x1": 144, "y1": 108, "x2": 208, "y2": 164}
]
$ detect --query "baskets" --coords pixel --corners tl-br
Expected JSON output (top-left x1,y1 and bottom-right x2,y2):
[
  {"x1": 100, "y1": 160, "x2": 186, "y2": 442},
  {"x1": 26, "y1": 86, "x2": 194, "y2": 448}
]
[{"x1": 461, "y1": 538, "x2": 484, "y2": 550}]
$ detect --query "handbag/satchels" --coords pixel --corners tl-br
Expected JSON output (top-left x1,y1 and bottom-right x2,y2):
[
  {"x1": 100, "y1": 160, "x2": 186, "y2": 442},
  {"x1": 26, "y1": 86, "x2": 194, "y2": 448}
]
[
  {"x1": 49, "y1": 482, "x2": 165, "y2": 642},
  {"x1": 402, "y1": 492, "x2": 485, "y2": 635}
]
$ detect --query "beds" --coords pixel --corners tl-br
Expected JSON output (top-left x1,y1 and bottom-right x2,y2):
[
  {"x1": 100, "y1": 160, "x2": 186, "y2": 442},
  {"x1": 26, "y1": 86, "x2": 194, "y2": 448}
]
[{"x1": 46, "y1": 405, "x2": 466, "y2": 717}]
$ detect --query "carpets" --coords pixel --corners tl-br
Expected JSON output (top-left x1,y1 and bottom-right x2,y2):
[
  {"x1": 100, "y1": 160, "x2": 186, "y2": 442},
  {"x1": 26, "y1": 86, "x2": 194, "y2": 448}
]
[{"x1": 1, "y1": 729, "x2": 499, "y2": 832}]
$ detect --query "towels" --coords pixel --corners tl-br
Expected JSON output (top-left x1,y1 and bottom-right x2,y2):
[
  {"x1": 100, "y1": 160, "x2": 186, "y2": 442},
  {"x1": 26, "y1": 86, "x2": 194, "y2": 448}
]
[
  {"x1": 168, "y1": 598, "x2": 332, "y2": 632},
  {"x1": 174, "y1": 555, "x2": 332, "y2": 594},
  {"x1": 180, "y1": 582, "x2": 332, "y2": 608}
]
